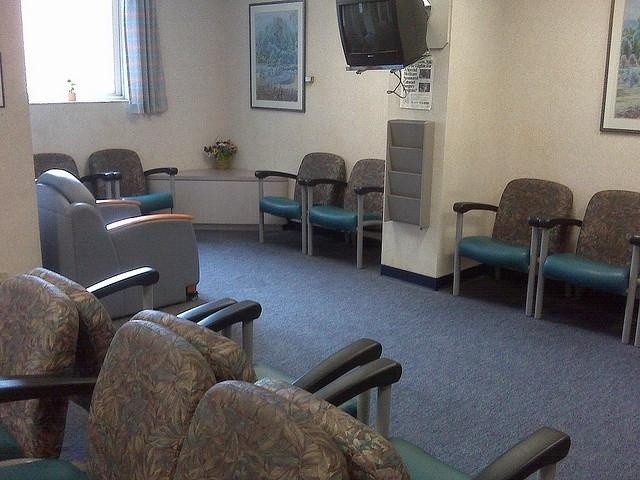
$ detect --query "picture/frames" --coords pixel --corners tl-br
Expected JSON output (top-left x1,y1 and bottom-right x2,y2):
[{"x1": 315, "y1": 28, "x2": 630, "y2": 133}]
[
  {"x1": 246, "y1": 2, "x2": 308, "y2": 115},
  {"x1": 599, "y1": 0, "x2": 640, "y2": 137}
]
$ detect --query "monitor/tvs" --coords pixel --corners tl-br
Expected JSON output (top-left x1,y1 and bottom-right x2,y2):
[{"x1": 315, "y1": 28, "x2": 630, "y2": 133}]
[{"x1": 336, "y1": 0, "x2": 429, "y2": 67}]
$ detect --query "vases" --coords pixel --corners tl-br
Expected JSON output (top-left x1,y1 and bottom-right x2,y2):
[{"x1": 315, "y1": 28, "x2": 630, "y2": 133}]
[{"x1": 68, "y1": 93, "x2": 77, "y2": 102}]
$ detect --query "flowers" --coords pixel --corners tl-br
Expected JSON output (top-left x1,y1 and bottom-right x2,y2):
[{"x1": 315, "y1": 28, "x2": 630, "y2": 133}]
[{"x1": 65, "y1": 77, "x2": 78, "y2": 94}]
